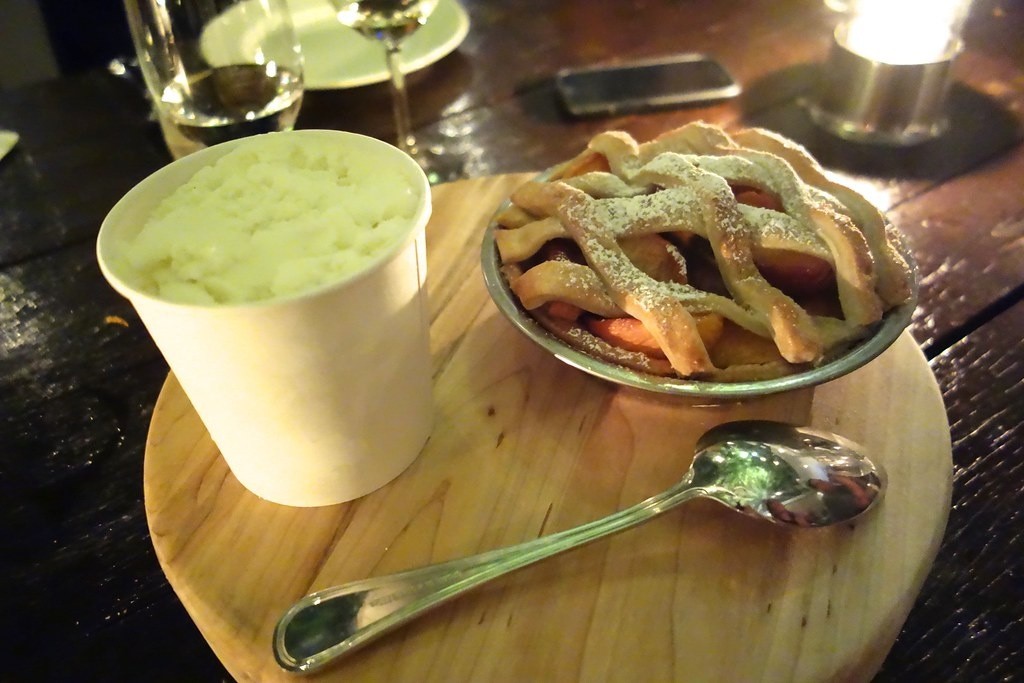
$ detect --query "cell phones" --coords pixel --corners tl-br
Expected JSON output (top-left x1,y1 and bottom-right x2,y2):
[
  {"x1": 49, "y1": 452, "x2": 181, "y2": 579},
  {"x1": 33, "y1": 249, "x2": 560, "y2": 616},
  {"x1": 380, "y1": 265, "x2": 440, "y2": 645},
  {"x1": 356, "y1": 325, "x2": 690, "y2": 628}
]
[{"x1": 554, "y1": 53, "x2": 742, "y2": 116}]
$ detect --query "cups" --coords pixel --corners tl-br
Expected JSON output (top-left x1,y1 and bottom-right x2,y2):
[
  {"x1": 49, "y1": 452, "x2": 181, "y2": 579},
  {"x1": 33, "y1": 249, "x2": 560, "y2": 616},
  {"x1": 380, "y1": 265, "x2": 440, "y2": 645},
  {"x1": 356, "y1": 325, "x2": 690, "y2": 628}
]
[
  {"x1": 125, "y1": 0, "x2": 305, "y2": 161},
  {"x1": 96, "y1": 127, "x2": 434, "y2": 507}
]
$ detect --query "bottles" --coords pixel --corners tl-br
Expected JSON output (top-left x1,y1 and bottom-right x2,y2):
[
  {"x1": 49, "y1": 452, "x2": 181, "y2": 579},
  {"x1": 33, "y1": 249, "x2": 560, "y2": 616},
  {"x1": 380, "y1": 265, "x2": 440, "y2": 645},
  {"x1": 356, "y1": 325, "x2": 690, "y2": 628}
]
[{"x1": 800, "y1": 21, "x2": 972, "y2": 145}]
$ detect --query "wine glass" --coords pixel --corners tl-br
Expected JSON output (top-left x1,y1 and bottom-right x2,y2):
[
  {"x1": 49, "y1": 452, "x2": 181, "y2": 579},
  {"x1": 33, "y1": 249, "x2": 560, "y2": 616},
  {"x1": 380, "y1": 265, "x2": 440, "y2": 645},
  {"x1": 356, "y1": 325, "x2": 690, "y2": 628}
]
[{"x1": 324, "y1": 1, "x2": 451, "y2": 190}]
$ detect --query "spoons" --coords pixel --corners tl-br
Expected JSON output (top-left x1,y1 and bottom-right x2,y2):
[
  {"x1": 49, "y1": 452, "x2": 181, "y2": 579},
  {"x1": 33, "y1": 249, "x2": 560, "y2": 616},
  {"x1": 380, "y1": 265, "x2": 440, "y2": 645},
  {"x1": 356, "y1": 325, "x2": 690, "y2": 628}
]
[{"x1": 272, "y1": 420, "x2": 889, "y2": 674}]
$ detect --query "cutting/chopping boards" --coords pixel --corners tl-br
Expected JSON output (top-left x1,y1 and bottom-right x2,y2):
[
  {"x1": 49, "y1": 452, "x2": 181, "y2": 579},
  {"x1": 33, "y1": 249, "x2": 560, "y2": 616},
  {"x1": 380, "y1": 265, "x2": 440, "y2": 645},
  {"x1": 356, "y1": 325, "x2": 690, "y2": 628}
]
[{"x1": 142, "y1": 175, "x2": 955, "y2": 683}]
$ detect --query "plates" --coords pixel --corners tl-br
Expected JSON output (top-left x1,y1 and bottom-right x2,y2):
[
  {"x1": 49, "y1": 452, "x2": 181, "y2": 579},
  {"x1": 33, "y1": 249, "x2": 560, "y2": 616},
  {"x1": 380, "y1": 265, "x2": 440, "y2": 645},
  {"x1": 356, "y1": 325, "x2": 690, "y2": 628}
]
[
  {"x1": 200, "y1": 0, "x2": 473, "y2": 90},
  {"x1": 477, "y1": 153, "x2": 918, "y2": 398}
]
